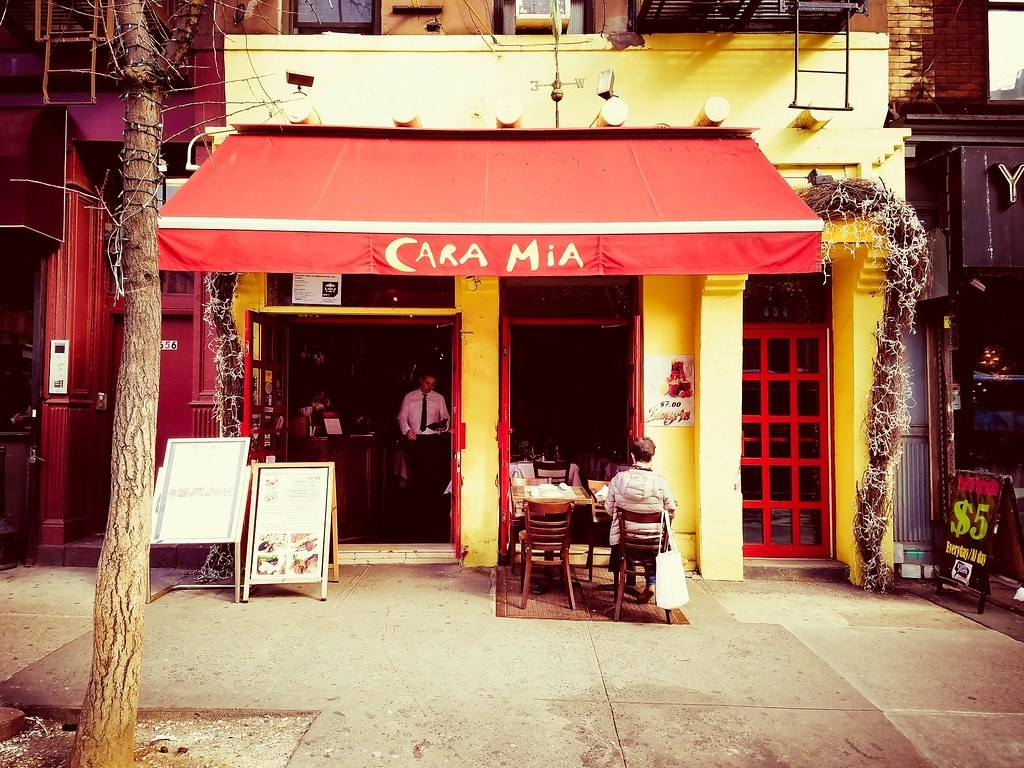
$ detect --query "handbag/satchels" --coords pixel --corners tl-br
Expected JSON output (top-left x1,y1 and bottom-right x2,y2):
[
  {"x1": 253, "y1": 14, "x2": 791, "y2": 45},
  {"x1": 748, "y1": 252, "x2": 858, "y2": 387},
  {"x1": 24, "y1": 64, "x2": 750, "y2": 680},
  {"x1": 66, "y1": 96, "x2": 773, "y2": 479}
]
[{"x1": 655, "y1": 510, "x2": 690, "y2": 610}]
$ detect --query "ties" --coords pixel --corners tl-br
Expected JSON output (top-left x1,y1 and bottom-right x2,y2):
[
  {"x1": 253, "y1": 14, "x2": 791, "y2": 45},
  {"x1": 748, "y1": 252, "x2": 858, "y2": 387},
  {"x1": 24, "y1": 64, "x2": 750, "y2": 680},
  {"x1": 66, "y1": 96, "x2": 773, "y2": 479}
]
[{"x1": 420, "y1": 393, "x2": 427, "y2": 432}]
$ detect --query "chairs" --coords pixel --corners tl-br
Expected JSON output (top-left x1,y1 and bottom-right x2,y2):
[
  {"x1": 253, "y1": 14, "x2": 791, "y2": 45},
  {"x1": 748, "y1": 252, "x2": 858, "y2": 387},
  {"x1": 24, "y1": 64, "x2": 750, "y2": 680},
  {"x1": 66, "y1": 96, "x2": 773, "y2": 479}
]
[
  {"x1": 614, "y1": 505, "x2": 674, "y2": 625},
  {"x1": 518, "y1": 498, "x2": 577, "y2": 611},
  {"x1": 509, "y1": 476, "x2": 553, "y2": 581},
  {"x1": 585, "y1": 479, "x2": 612, "y2": 580},
  {"x1": 532, "y1": 460, "x2": 571, "y2": 485}
]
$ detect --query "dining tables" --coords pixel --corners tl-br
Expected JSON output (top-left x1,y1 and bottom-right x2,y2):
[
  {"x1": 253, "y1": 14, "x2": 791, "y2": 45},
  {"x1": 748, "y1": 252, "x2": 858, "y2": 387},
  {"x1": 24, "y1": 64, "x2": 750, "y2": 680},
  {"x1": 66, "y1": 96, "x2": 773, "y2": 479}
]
[
  {"x1": 604, "y1": 463, "x2": 630, "y2": 480},
  {"x1": 509, "y1": 485, "x2": 593, "y2": 592},
  {"x1": 590, "y1": 456, "x2": 625, "y2": 479},
  {"x1": 589, "y1": 486, "x2": 610, "y2": 505},
  {"x1": 510, "y1": 461, "x2": 582, "y2": 489}
]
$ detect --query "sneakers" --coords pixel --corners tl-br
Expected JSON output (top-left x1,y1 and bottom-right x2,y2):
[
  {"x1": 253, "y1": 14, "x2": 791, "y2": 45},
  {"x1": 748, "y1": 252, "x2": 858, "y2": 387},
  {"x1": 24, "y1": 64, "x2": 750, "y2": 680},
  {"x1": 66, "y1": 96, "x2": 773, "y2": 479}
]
[{"x1": 636, "y1": 585, "x2": 655, "y2": 604}]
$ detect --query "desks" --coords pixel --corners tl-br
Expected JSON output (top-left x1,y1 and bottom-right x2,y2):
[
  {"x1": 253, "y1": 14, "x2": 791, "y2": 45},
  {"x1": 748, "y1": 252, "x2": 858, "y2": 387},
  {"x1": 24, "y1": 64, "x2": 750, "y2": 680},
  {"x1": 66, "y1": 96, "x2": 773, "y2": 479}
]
[{"x1": 288, "y1": 431, "x2": 387, "y2": 544}]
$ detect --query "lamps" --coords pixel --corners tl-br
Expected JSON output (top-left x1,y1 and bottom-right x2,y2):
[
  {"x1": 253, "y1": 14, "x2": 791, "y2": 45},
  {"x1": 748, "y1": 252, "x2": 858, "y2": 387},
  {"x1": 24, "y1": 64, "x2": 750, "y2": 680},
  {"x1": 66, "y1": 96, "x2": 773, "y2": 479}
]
[{"x1": 597, "y1": 69, "x2": 619, "y2": 100}]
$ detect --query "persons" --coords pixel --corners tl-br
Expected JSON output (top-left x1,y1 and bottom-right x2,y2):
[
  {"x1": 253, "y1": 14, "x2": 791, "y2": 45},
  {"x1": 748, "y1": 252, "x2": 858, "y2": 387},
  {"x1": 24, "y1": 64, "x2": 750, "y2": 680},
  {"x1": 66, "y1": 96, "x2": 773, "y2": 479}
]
[
  {"x1": 604, "y1": 436, "x2": 674, "y2": 602},
  {"x1": 399, "y1": 369, "x2": 451, "y2": 441}
]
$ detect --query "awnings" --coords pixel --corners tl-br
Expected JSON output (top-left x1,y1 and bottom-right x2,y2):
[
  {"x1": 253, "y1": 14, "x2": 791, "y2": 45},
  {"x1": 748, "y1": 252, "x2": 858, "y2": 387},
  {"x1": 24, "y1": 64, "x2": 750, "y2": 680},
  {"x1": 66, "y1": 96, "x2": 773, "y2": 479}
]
[{"x1": 152, "y1": 119, "x2": 823, "y2": 274}]
